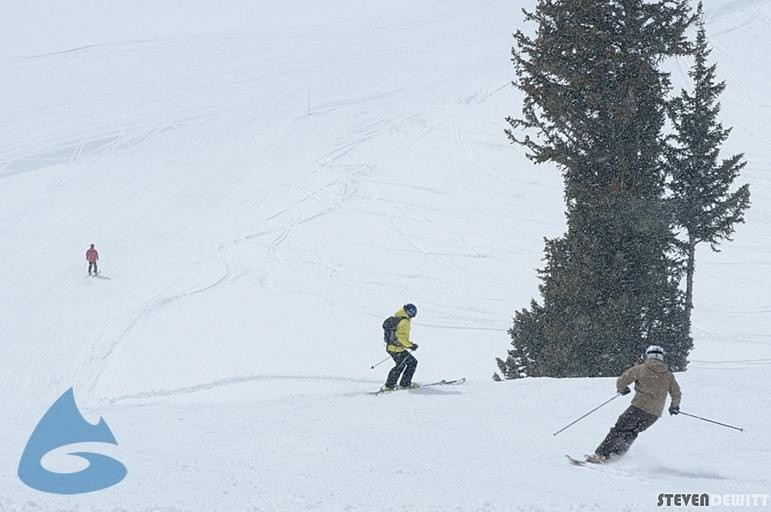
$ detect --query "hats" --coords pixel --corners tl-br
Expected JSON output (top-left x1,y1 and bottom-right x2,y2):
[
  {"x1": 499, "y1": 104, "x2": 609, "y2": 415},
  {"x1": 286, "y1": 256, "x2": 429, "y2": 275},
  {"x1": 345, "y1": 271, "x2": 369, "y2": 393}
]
[{"x1": 403, "y1": 304, "x2": 417, "y2": 317}]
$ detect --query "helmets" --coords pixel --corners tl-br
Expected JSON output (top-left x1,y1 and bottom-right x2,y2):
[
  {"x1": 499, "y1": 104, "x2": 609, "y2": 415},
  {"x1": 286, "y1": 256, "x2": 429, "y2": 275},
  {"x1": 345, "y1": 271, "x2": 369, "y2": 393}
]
[{"x1": 645, "y1": 345, "x2": 666, "y2": 361}]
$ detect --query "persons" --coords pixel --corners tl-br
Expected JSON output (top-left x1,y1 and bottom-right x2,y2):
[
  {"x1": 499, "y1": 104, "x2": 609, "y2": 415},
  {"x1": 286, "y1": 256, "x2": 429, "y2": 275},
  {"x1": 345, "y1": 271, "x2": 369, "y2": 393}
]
[
  {"x1": 383, "y1": 303, "x2": 419, "y2": 392},
  {"x1": 85, "y1": 244, "x2": 99, "y2": 273},
  {"x1": 584, "y1": 344, "x2": 682, "y2": 463}
]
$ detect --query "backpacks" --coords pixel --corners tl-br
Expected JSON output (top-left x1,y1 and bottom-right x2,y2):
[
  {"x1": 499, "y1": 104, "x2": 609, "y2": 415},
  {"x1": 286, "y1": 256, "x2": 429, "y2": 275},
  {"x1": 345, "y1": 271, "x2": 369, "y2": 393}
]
[{"x1": 382, "y1": 316, "x2": 409, "y2": 346}]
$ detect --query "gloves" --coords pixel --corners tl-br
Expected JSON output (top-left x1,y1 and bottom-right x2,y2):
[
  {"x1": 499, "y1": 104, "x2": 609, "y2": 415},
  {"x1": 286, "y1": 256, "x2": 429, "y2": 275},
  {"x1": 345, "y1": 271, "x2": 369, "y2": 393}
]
[
  {"x1": 410, "y1": 344, "x2": 418, "y2": 351},
  {"x1": 669, "y1": 406, "x2": 680, "y2": 416},
  {"x1": 620, "y1": 387, "x2": 630, "y2": 396}
]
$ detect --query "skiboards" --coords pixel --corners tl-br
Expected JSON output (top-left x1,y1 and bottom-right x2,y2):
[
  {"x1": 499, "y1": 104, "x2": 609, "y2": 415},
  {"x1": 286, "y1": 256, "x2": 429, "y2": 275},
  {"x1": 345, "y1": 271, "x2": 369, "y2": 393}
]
[
  {"x1": 366, "y1": 378, "x2": 465, "y2": 394},
  {"x1": 566, "y1": 453, "x2": 669, "y2": 483},
  {"x1": 84, "y1": 271, "x2": 101, "y2": 277}
]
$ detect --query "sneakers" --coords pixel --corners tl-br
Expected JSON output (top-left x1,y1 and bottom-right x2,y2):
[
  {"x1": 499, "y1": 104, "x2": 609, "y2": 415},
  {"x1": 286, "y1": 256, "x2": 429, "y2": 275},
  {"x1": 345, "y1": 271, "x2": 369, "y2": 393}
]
[
  {"x1": 400, "y1": 382, "x2": 420, "y2": 389},
  {"x1": 586, "y1": 454, "x2": 609, "y2": 464},
  {"x1": 383, "y1": 386, "x2": 394, "y2": 391}
]
